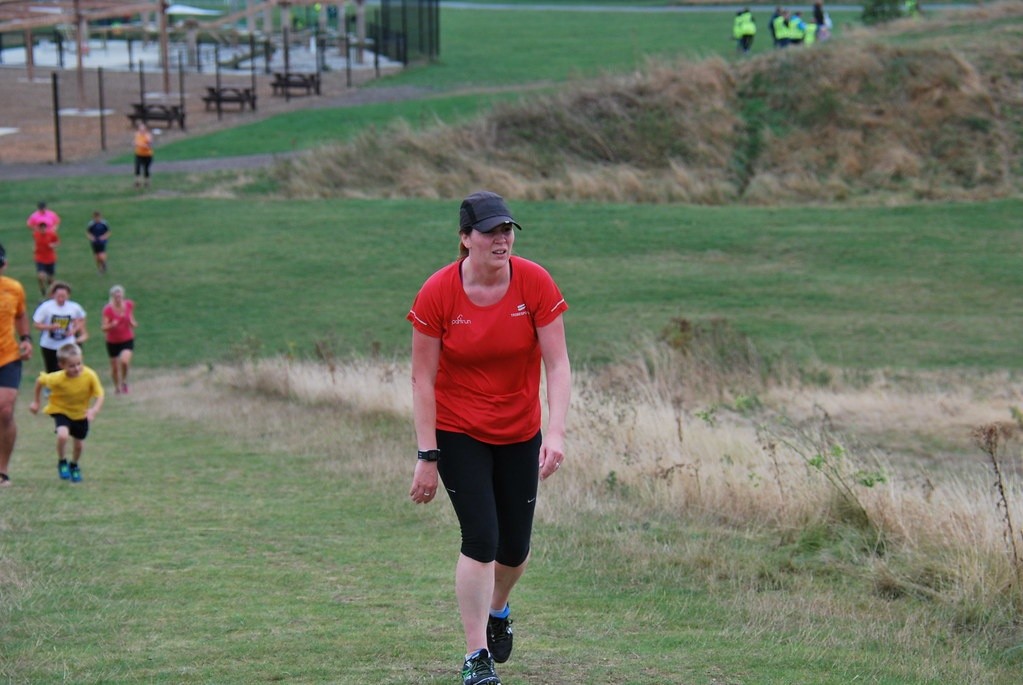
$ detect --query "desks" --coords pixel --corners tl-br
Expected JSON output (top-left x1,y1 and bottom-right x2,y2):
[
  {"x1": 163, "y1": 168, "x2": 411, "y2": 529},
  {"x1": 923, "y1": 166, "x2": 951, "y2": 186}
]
[
  {"x1": 131, "y1": 102, "x2": 184, "y2": 128},
  {"x1": 271, "y1": 71, "x2": 320, "y2": 96},
  {"x1": 206, "y1": 84, "x2": 255, "y2": 109}
]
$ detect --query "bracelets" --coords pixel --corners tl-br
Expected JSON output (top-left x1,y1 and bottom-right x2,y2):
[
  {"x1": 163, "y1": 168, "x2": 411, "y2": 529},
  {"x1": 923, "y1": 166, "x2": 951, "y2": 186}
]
[{"x1": 19, "y1": 335, "x2": 32, "y2": 343}]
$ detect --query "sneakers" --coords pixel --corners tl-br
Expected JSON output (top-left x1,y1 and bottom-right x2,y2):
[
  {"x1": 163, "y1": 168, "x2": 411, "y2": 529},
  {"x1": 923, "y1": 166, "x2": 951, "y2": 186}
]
[
  {"x1": 486, "y1": 614, "x2": 513, "y2": 663},
  {"x1": 462, "y1": 649, "x2": 501, "y2": 685}
]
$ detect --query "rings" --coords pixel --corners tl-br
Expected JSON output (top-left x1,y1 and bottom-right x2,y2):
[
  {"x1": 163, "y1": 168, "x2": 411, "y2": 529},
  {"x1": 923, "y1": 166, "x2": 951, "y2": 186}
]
[
  {"x1": 424, "y1": 493, "x2": 429, "y2": 496},
  {"x1": 556, "y1": 463, "x2": 560, "y2": 468}
]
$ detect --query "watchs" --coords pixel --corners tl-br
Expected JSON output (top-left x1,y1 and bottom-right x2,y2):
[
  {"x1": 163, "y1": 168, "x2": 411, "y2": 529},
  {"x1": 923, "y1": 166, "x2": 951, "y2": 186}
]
[{"x1": 417, "y1": 448, "x2": 440, "y2": 463}]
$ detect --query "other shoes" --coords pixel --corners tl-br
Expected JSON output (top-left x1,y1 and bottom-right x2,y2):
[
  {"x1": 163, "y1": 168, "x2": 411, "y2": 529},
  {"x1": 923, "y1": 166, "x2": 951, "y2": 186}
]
[
  {"x1": 0, "y1": 473, "x2": 9, "y2": 483},
  {"x1": 122, "y1": 383, "x2": 128, "y2": 393},
  {"x1": 69, "y1": 463, "x2": 80, "y2": 481},
  {"x1": 59, "y1": 459, "x2": 68, "y2": 479}
]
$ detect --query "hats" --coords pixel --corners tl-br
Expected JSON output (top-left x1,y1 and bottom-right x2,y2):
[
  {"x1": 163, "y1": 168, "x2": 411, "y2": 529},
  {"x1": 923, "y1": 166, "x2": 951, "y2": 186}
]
[{"x1": 460, "y1": 191, "x2": 522, "y2": 233}]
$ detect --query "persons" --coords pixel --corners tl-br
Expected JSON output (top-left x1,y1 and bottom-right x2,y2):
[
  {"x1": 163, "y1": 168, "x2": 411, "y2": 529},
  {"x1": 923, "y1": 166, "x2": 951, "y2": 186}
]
[
  {"x1": 409, "y1": 191, "x2": 573, "y2": 684},
  {"x1": 131, "y1": 123, "x2": 154, "y2": 188},
  {"x1": 33, "y1": 280, "x2": 89, "y2": 399},
  {"x1": 0, "y1": 243, "x2": 33, "y2": 488},
  {"x1": 27, "y1": 201, "x2": 60, "y2": 247},
  {"x1": 101, "y1": 285, "x2": 138, "y2": 396},
  {"x1": 32, "y1": 222, "x2": 61, "y2": 297},
  {"x1": 29, "y1": 344, "x2": 105, "y2": 483},
  {"x1": 731, "y1": 0, "x2": 832, "y2": 58},
  {"x1": 85, "y1": 211, "x2": 112, "y2": 273}
]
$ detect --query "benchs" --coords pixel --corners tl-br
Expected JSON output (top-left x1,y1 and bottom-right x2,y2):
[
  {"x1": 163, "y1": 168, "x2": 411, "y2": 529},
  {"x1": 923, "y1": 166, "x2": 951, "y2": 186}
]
[
  {"x1": 204, "y1": 95, "x2": 258, "y2": 103},
  {"x1": 126, "y1": 113, "x2": 185, "y2": 122},
  {"x1": 271, "y1": 80, "x2": 322, "y2": 87}
]
